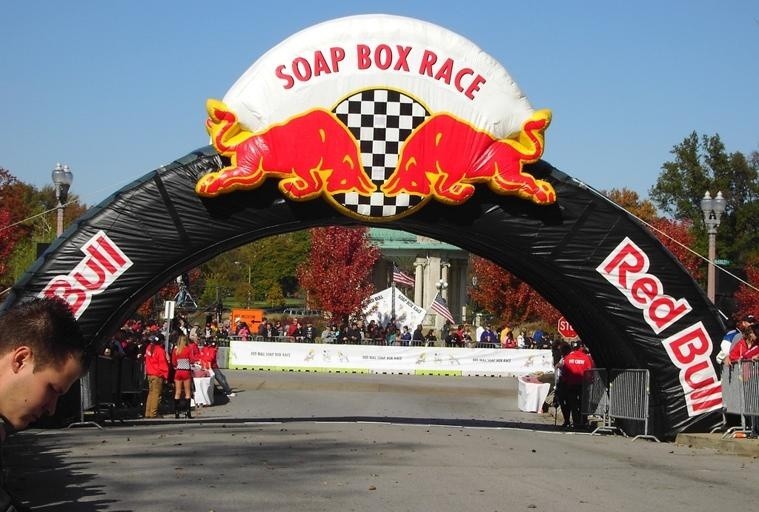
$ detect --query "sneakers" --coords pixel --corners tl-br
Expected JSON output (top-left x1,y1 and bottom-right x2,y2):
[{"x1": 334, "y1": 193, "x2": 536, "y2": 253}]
[{"x1": 145, "y1": 415, "x2": 163, "y2": 418}]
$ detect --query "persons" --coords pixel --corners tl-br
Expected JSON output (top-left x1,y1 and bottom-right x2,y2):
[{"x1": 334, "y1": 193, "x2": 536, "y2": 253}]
[
  {"x1": 322, "y1": 320, "x2": 437, "y2": 347},
  {"x1": 106, "y1": 317, "x2": 313, "y2": 371},
  {"x1": 144, "y1": 334, "x2": 170, "y2": 418},
  {"x1": 0, "y1": 296, "x2": 97, "y2": 445},
  {"x1": 441, "y1": 320, "x2": 595, "y2": 431},
  {"x1": 170, "y1": 333, "x2": 196, "y2": 419},
  {"x1": 203, "y1": 342, "x2": 239, "y2": 398},
  {"x1": 716, "y1": 313, "x2": 758, "y2": 440}
]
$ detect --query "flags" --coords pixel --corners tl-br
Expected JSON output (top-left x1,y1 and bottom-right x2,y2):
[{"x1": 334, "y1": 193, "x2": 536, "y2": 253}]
[
  {"x1": 393, "y1": 265, "x2": 415, "y2": 286},
  {"x1": 431, "y1": 292, "x2": 456, "y2": 324}
]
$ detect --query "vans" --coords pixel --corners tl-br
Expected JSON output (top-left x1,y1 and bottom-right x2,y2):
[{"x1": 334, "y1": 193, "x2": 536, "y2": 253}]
[{"x1": 283, "y1": 308, "x2": 321, "y2": 318}]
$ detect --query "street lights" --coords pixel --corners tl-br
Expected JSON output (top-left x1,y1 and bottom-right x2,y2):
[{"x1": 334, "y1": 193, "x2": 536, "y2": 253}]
[
  {"x1": 52, "y1": 163, "x2": 72, "y2": 238},
  {"x1": 700, "y1": 190, "x2": 727, "y2": 304},
  {"x1": 234, "y1": 261, "x2": 251, "y2": 309},
  {"x1": 435, "y1": 262, "x2": 451, "y2": 296},
  {"x1": 412, "y1": 256, "x2": 432, "y2": 309}
]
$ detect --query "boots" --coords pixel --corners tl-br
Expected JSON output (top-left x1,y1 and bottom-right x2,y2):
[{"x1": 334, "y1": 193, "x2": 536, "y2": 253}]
[
  {"x1": 175, "y1": 399, "x2": 180, "y2": 418},
  {"x1": 185, "y1": 398, "x2": 194, "y2": 419}
]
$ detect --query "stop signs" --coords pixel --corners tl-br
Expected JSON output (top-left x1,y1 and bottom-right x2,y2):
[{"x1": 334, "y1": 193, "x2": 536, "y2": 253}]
[{"x1": 558, "y1": 316, "x2": 579, "y2": 339}]
